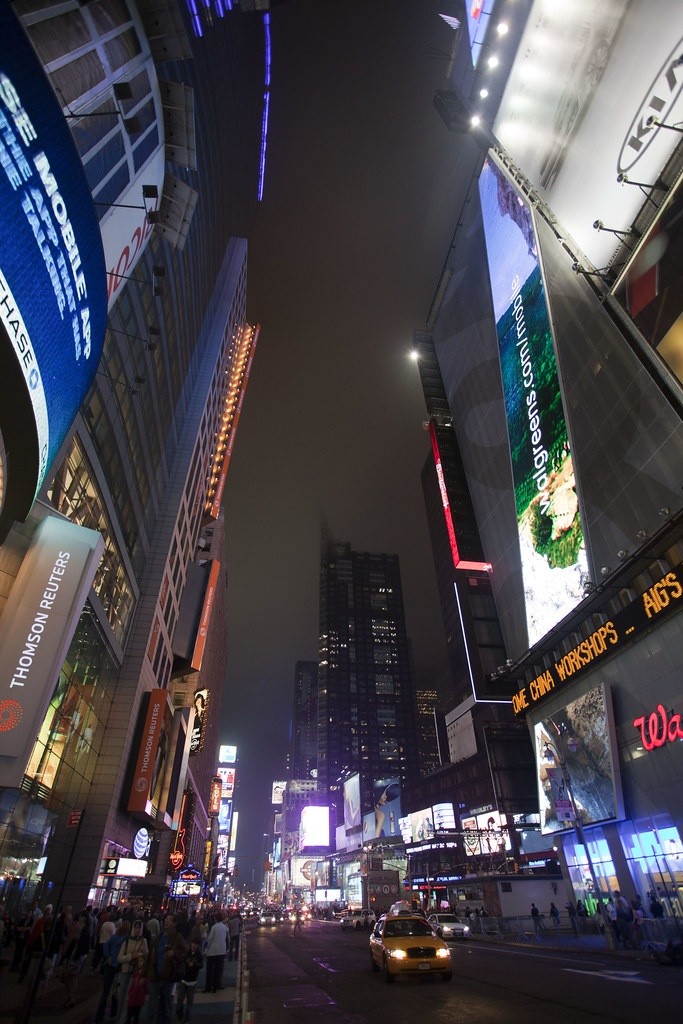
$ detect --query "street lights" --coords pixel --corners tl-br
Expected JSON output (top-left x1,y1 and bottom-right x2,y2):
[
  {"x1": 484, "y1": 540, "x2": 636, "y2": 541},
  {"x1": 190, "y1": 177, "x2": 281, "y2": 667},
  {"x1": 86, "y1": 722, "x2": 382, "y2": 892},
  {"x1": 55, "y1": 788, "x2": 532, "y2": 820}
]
[{"x1": 542, "y1": 740, "x2": 619, "y2": 951}]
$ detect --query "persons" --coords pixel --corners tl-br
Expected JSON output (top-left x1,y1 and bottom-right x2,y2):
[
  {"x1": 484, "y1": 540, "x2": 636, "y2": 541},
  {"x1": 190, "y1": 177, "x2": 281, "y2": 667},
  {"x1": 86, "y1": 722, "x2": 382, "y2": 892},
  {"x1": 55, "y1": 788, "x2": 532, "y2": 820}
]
[
  {"x1": 531, "y1": 902, "x2": 542, "y2": 933},
  {"x1": 294, "y1": 911, "x2": 302, "y2": 933},
  {"x1": 0, "y1": 901, "x2": 241, "y2": 1024},
  {"x1": 596, "y1": 890, "x2": 664, "y2": 950},
  {"x1": 242, "y1": 899, "x2": 349, "y2": 929},
  {"x1": 349, "y1": 907, "x2": 386, "y2": 930},
  {"x1": 549, "y1": 902, "x2": 560, "y2": 928},
  {"x1": 373, "y1": 780, "x2": 400, "y2": 838},
  {"x1": 425, "y1": 906, "x2": 456, "y2": 920},
  {"x1": 465, "y1": 906, "x2": 488, "y2": 930},
  {"x1": 565, "y1": 900, "x2": 588, "y2": 930}
]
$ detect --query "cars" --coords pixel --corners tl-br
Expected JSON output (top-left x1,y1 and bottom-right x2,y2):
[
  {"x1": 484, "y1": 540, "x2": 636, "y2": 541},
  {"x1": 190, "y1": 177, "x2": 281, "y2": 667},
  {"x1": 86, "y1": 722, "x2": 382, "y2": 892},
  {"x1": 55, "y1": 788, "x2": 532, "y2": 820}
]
[
  {"x1": 426, "y1": 914, "x2": 470, "y2": 942},
  {"x1": 340, "y1": 909, "x2": 377, "y2": 931},
  {"x1": 242, "y1": 905, "x2": 311, "y2": 926}
]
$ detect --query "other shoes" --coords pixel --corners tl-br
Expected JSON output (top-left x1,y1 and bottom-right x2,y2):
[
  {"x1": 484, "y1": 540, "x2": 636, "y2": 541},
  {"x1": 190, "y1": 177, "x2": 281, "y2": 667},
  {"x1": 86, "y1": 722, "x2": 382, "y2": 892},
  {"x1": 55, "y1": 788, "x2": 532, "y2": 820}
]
[
  {"x1": 178, "y1": 1012, "x2": 184, "y2": 1020},
  {"x1": 61, "y1": 996, "x2": 81, "y2": 1007},
  {"x1": 202, "y1": 988, "x2": 216, "y2": 993},
  {"x1": 184, "y1": 1013, "x2": 193, "y2": 1022},
  {"x1": 9, "y1": 967, "x2": 16, "y2": 972},
  {"x1": 90, "y1": 966, "x2": 94, "y2": 978},
  {"x1": 17, "y1": 975, "x2": 24, "y2": 985}
]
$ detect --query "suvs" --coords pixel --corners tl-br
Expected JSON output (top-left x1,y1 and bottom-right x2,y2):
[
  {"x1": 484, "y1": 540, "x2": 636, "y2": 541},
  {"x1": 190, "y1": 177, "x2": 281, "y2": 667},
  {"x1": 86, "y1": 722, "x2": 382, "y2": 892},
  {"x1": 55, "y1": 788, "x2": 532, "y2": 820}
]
[{"x1": 369, "y1": 914, "x2": 453, "y2": 981}]
